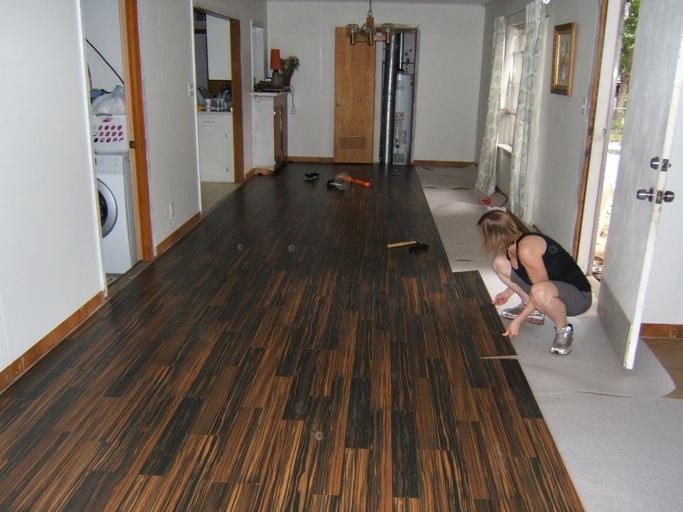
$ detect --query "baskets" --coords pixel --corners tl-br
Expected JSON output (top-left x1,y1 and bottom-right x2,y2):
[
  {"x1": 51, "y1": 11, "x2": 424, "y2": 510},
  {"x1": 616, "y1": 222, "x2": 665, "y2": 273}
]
[{"x1": 90, "y1": 114, "x2": 129, "y2": 154}]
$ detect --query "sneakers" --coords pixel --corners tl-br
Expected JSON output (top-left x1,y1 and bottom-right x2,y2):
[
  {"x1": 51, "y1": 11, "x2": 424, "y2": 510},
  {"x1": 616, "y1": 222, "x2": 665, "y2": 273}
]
[
  {"x1": 502, "y1": 303, "x2": 545, "y2": 325},
  {"x1": 550, "y1": 324, "x2": 573, "y2": 354}
]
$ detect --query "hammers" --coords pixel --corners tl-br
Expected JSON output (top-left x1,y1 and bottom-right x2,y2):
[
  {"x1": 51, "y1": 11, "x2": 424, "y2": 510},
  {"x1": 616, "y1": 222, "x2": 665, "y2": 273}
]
[{"x1": 386, "y1": 240, "x2": 428, "y2": 249}]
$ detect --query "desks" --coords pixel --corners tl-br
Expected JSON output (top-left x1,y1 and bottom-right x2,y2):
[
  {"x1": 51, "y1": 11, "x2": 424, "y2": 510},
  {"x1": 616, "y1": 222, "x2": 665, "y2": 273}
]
[{"x1": 252, "y1": 92, "x2": 287, "y2": 175}]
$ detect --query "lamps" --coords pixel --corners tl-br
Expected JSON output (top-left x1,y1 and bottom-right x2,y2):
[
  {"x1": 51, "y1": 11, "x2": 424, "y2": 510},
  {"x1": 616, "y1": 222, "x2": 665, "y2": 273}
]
[{"x1": 345, "y1": 0, "x2": 391, "y2": 45}]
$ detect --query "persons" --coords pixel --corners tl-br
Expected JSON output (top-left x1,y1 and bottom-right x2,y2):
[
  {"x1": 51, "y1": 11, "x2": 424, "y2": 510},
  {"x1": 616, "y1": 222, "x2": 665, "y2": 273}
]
[{"x1": 478, "y1": 211, "x2": 593, "y2": 354}]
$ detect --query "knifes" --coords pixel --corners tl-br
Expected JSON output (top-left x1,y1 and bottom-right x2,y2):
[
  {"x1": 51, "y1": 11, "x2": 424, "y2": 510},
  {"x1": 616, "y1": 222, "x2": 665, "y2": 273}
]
[{"x1": 197, "y1": 84, "x2": 229, "y2": 98}]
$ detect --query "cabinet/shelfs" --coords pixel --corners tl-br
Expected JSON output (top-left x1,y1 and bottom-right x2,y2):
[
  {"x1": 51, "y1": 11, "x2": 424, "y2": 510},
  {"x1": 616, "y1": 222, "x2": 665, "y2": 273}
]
[{"x1": 199, "y1": 116, "x2": 235, "y2": 183}]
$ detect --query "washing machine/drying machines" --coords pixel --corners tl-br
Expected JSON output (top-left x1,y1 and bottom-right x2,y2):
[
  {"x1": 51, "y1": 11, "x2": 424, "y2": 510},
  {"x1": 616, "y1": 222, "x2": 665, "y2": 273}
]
[{"x1": 92, "y1": 153, "x2": 140, "y2": 275}]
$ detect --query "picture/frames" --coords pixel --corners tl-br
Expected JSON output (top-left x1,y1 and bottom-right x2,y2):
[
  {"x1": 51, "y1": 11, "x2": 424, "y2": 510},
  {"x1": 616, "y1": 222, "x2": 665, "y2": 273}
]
[{"x1": 550, "y1": 22, "x2": 576, "y2": 95}]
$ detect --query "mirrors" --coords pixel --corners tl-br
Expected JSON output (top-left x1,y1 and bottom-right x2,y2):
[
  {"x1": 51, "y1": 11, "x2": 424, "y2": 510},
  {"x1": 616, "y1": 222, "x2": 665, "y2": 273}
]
[{"x1": 250, "y1": 19, "x2": 268, "y2": 91}]
